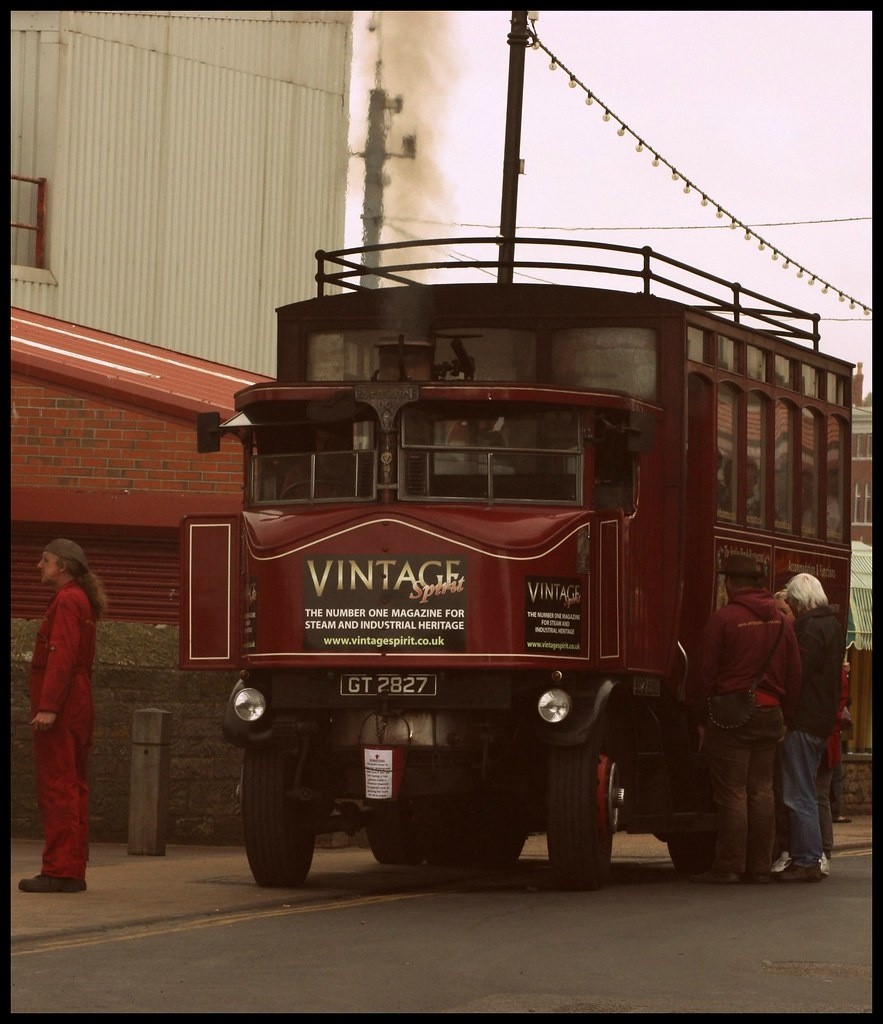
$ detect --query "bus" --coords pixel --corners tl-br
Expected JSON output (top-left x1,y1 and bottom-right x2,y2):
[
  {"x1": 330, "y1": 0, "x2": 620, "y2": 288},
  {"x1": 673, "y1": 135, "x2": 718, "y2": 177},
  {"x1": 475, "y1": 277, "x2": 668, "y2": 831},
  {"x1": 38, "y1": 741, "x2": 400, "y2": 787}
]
[{"x1": 178, "y1": 11, "x2": 857, "y2": 895}]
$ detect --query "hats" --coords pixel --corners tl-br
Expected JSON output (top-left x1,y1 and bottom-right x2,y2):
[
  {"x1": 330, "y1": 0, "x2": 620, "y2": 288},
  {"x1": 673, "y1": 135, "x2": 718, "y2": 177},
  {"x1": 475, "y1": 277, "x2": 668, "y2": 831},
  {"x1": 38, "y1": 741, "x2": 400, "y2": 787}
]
[
  {"x1": 44, "y1": 538, "x2": 90, "y2": 572},
  {"x1": 719, "y1": 554, "x2": 765, "y2": 577}
]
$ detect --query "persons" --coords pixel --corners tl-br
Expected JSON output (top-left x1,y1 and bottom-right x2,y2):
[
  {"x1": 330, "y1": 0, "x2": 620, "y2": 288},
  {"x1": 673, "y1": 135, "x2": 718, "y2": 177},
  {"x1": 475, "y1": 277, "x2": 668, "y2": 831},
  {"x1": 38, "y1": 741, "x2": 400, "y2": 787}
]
[
  {"x1": 280, "y1": 423, "x2": 370, "y2": 499},
  {"x1": 715, "y1": 447, "x2": 726, "y2": 510},
  {"x1": 722, "y1": 456, "x2": 780, "y2": 521},
  {"x1": 780, "y1": 464, "x2": 841, "y2": 532},
  {"x1": 698, "y1": 554, "x2": 803, "y2": 885},
  {"x1": 18, "y1": 539, "x2": 106, "y2": 894},
  {"x1": 772, "y1": 572, "x2": 853, "y2": 879}
]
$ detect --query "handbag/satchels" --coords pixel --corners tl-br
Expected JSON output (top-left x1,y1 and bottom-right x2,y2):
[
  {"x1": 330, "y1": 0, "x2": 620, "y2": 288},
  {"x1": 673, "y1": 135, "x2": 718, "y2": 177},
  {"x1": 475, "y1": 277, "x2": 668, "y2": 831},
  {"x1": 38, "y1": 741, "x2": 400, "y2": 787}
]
[{"x1": 707, "y1": 689, "x2": 758, "y2": 732}]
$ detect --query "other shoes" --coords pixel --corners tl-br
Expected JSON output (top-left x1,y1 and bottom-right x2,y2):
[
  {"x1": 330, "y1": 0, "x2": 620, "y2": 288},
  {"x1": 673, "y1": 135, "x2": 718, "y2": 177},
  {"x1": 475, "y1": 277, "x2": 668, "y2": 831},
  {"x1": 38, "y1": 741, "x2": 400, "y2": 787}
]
[
  {"x1": 19, "y1": 874, "x2": 78, "y2": 892},
  {"x1": 76, "y1": 878, "x2": 87, "y2": 890},
  {"x1": 752, "y1": 871, "x2": 769, "y2": 883},
  {"x1": 776, "y1": 863, "x2": 821, "y2": 882},
  {"x1": 833, "y1": 818, "x2": 852, "y2": 822},
  {"x1": 690, "y1": 869, "x2": 738, "y2": 882}
]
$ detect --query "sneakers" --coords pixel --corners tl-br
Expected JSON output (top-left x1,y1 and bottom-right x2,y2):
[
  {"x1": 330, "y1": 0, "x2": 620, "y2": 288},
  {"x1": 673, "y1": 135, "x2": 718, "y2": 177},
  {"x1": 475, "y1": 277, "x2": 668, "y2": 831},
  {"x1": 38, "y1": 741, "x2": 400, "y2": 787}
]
[
  {"x1": 771, "y1": 851, "x2": 793, "y2": 872},
  {"x1": 818, "y1": 853, "x2": 830, "y2": 875}
]
